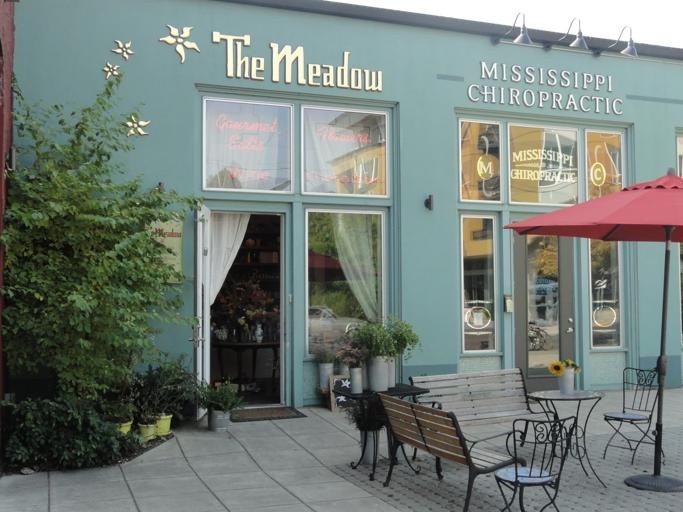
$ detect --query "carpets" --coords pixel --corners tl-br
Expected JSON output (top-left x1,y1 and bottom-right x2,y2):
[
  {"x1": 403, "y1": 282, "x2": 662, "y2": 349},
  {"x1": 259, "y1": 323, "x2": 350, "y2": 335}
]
[{"x1": 230, "y1": 408, "x2": 307, "y2": 425}]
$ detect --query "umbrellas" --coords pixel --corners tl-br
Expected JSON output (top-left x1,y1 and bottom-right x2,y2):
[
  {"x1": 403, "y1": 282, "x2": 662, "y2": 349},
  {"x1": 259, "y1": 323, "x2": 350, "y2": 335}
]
[{"x1": 502, "y1": 167, "x2": 683, "y2": 493}]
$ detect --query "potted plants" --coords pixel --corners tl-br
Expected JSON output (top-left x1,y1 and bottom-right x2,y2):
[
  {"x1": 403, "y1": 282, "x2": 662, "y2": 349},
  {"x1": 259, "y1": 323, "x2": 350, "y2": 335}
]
[
  {"x1": 308, "y1": 315, "x2": 419, "y2": 394},
  {"x1": 197, "y1": 378, "x2": 245, "y2": 432},
  {"x1": 101, "y1": 352, "x2": 197, "y2": 439}
]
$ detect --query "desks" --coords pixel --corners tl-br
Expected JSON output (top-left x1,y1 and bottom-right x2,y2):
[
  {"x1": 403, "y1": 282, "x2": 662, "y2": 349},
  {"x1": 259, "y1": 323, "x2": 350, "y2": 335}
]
[
  {"x1": 527, "y1": 390, "x2": 607, "y2": 488},
  {"x1": 332, "y1": 383, "x2": 429, "y2": 481},
  {"x1": 212, "y1": 343, "x2": 280, "y2": 385}
]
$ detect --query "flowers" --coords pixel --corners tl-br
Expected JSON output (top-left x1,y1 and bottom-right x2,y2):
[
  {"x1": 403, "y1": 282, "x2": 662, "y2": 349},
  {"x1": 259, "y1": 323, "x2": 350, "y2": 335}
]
[
  {"x1": 548, "y1": 359, "x2": 582, "y2": 377},
  {"x1": 208, "y1": 272, "x2": 280, "y2": 342},
  {"x1": 333, "y1": 379, "x2": 388, "y2": 431}
]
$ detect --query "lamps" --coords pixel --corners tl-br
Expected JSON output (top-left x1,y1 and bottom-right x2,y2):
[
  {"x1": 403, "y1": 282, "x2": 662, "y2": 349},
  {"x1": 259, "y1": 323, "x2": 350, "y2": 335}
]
[{"x1": 504, "y1": 13, "x2": 638, "y2": 60}]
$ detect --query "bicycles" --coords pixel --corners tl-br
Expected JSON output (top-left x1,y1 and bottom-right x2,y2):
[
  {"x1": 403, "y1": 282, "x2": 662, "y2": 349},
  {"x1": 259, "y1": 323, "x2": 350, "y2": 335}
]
[{"x1": 528, "y1": 320, "x2": 554, "y2": 351}]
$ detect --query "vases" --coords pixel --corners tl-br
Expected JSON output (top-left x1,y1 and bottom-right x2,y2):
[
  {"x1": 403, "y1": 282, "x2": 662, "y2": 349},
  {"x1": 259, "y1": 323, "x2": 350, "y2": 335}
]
[
  {"x1": 252, "y1": 321, "x2": 266, "y2": 344},
  {"x1": 558, "y1": 368, "x2": 575, "y2": 393},
  {"x1": 360, "y1": 429, "x2": 379, "y2": 467}
]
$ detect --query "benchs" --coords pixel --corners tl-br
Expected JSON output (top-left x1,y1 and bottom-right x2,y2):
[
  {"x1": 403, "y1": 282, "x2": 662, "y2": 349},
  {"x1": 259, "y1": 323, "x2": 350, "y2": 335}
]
[
  {"x1": 410, "y1": 368, "x2": 558, "y2": 461},
  {"x1": 379, "y1": 393, "x2": 527, "y2": 512}
]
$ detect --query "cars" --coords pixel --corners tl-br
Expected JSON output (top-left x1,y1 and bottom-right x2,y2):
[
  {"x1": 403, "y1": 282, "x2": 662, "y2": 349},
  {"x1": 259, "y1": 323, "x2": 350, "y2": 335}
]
[
  {"x1": 465, "y1": 300, "x2": 493, "y2": 337},
  {"x1": 309, "y1": 305, "x2": 367, "y2": 339},
  {"x1": 530, "y1": 282, "x2": 558, "y2": 309}
]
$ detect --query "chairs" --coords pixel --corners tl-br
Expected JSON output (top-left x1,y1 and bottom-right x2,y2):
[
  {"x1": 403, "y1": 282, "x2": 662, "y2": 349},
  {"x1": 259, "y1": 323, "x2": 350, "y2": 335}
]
[
  {"x1": 602, "y1": 366, "x2": 665, "y2": 465},
  {"x1": 494, "y1": 416, "x2": 577, "y2": 512}
]
